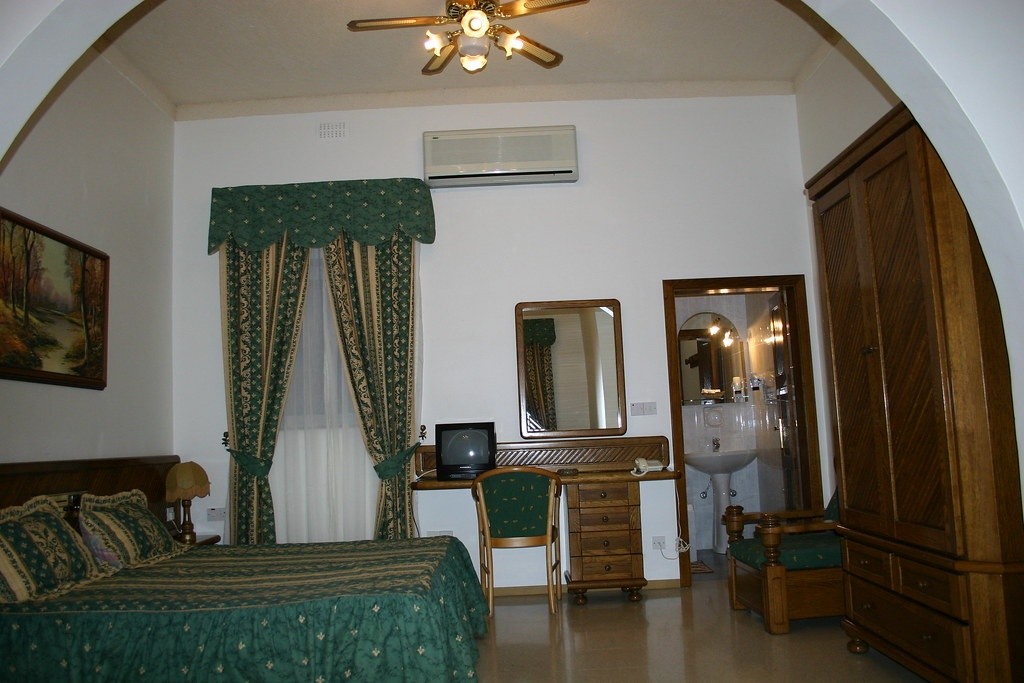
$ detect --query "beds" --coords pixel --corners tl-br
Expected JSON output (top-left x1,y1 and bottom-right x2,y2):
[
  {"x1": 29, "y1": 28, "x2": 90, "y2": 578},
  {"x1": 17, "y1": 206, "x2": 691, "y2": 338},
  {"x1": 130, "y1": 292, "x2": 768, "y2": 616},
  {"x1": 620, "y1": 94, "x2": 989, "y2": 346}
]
[{"x1": 0, "y1": 448, "x2": 491, "y2": 683}]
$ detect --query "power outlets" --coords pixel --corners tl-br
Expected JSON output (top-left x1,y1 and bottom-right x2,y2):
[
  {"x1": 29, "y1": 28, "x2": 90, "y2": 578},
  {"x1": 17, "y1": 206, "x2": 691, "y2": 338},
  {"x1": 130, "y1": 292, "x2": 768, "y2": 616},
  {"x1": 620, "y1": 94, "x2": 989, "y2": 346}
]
[
  {"x1": 652, "y1": 536, "x2": 666, "y2": 550},
  {"x1": 691, "y1": 559, "x2": 713, "y2": 573}
]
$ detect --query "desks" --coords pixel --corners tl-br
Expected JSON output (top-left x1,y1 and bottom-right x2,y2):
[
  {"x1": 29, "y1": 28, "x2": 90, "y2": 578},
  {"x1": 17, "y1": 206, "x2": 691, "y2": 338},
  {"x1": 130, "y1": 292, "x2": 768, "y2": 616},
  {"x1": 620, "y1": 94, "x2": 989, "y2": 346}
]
[{"x1": 410, "y1": 436, "x2": 675, "y2": 488}]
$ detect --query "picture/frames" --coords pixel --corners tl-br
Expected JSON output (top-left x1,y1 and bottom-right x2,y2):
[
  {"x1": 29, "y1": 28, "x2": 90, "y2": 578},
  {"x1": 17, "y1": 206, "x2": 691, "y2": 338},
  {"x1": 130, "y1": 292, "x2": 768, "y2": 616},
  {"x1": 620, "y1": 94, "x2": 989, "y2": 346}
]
[{"x1": 0, "y1": 206, "x2": 111, "y2": 391}]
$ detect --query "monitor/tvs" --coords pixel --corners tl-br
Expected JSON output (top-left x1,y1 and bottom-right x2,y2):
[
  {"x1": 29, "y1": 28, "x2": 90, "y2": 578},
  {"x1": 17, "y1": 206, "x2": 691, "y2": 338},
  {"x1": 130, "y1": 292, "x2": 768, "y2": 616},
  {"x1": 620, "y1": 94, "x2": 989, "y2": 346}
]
[{"x1": 435, "y1": 422, "x2": 497, "y2": 481}]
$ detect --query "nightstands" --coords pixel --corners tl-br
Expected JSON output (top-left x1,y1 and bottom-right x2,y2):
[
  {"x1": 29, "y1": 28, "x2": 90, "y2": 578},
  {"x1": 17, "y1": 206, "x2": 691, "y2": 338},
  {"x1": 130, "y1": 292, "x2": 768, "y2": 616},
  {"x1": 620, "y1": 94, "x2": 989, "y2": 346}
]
[{"x1": 194, "y1": 534, "x2": 222, "y2": 544}]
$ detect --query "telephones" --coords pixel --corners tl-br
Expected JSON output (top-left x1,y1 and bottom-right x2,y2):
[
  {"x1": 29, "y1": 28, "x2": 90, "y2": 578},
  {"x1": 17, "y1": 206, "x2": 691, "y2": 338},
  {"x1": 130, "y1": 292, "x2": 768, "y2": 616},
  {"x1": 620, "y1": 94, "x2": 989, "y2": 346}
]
[{"x1": 635, "y1": 457, "x2": 664, "y2": 472}]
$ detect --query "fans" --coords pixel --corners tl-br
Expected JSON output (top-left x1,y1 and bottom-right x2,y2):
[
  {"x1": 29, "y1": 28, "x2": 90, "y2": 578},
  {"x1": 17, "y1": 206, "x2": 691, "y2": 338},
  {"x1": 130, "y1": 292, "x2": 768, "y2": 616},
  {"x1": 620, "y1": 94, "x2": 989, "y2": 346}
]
[{"x1": 346, "y1": 0, "x2": 589, "y2": 75}]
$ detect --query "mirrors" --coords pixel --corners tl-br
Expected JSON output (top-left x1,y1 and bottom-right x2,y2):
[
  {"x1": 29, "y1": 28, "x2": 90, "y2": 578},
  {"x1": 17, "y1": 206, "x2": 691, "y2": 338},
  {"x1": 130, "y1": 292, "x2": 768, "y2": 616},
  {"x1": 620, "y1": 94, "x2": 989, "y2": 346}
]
[
  {"x1": 677, "y1": 311, "x2": 748, "y2": 407},
  {"x1": 514, "y1": 296, "x2": 625, "y2": 441}
]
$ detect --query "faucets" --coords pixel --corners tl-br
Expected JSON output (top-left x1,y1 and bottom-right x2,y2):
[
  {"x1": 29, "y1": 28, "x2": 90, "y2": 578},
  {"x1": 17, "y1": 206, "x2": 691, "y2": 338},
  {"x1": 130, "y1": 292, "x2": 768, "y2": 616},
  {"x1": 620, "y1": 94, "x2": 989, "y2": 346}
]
[{"x1": 712, "y1": 438, "x2": 720, "y2": 452}]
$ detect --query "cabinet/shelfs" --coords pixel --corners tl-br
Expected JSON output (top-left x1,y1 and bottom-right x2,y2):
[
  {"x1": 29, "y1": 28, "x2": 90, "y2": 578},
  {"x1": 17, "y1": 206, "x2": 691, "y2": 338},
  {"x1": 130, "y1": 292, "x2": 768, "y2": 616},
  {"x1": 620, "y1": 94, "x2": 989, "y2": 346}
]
[
  {"x1": 565, "y1": 484, "x2": 645, "y2": 606},
  {"x1": 806, "y1": 100, "x2": 1024, "y2": 683}
]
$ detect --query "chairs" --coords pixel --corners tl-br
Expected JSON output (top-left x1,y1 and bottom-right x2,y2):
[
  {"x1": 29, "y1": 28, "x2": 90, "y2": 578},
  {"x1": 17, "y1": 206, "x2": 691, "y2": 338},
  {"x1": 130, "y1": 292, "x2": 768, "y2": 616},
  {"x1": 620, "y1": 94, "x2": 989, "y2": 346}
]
[
  {"x1": 471, "y1": 467, "x2": 563, "y2": 618},
  {"x1": 723, "y1": 484, "x2": 845, "y2": 636}
]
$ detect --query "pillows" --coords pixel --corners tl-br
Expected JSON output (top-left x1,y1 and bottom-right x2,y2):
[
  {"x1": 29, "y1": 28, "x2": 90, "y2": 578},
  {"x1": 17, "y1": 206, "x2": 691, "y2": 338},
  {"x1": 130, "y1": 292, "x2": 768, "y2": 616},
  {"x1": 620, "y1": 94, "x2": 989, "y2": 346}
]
[
  {"x1": 0, "y1": 495, "x2": 122, "y2": 610},
  {"x1": 77, "y1": 488, "x2": 194, "y2": 578}
]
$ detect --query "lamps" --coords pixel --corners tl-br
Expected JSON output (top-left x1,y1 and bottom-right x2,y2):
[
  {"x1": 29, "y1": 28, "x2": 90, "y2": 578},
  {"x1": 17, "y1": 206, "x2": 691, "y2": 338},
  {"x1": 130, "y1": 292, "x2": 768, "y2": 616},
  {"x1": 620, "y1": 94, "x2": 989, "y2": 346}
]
[
  {"x1": 166, "y1": 461, "x2": 212, "y2": 543},
  {"x1": 424, "y1": 10, "x2": 523, "y2": 73}
]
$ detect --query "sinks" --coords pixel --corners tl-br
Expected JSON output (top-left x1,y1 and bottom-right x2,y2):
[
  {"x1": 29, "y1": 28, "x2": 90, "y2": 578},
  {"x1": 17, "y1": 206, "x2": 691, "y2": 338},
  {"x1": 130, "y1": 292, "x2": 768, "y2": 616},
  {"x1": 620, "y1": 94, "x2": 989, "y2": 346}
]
[{"x1": 684, "y1": 449, "x2": 756, "y2": 475}]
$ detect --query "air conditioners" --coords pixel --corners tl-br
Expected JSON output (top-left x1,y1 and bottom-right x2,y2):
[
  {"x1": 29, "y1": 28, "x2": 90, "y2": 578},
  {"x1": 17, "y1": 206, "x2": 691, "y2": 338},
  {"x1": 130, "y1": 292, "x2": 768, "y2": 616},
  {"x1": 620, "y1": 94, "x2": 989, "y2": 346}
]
[{"x1": 424, "y1": 124, "x2": 579, "y2": 190}]
